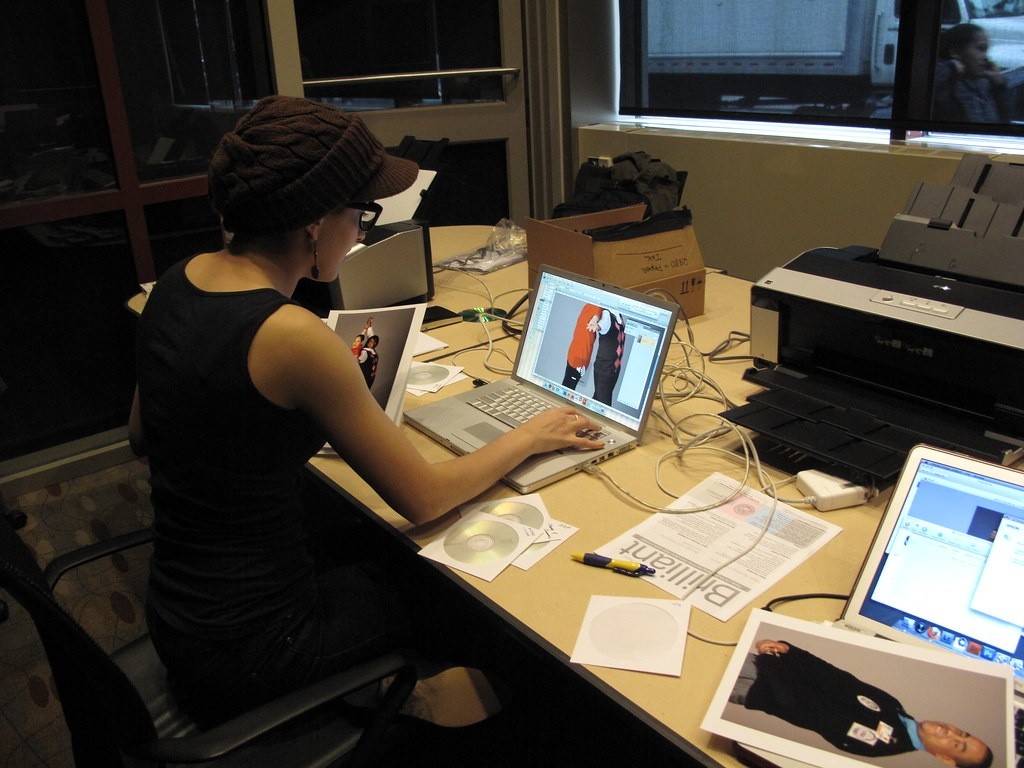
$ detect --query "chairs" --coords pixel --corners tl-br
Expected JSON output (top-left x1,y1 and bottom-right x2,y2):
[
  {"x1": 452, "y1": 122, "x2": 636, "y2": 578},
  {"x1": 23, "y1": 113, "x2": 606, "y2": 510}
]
[{"x1": 0, "y1": 510, "x2": 420, "y2": 768}]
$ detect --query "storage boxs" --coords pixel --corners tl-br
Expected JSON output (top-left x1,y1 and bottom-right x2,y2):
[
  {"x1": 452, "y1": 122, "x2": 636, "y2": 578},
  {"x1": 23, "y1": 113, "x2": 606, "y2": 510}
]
[{"x1": 525, "y1": 204, "x2": 705, "y2": 321}]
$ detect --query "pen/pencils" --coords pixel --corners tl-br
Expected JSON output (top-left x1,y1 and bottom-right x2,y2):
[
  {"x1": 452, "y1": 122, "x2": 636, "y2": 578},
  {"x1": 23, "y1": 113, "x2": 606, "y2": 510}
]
[{"x1": 567, "y1": 546, "x2": 661, "y2": 581}]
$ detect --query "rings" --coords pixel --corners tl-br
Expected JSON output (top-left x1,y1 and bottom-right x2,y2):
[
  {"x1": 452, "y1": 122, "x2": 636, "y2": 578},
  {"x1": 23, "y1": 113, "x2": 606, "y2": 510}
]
[{"x1": 574, "y1": 414, "x2": 578, "y2": 421}]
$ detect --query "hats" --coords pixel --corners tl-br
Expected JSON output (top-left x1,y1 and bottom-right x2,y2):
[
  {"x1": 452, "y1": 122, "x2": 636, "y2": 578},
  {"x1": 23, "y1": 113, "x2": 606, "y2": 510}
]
[{"x1": 206, "y1": 95, "x2": 419, "y2": 235}]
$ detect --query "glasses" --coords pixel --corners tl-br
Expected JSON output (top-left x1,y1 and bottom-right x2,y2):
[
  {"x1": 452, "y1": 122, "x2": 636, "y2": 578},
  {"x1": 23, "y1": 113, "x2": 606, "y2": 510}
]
[{"x1": 346, "y1": 202, "x2": 384, "y2": 231}]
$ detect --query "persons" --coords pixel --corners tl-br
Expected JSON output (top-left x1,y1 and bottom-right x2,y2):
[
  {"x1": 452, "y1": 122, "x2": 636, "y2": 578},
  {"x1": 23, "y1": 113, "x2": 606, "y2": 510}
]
[
  {"x1": 351, "y1": 318, "x2": 379, "y2": 390},
  {"x1": 728, "y1": 640, "x2": 994, "y2": 768},
  {"x1": 127, "y1": 96, "x2": 605, "y2": 745},
  {"x1": 562, "y1": 303, "x2": 625, "y2": 406},
  {"x1": 930, "y1": 23, "x2": 1012, "y2": 125}
]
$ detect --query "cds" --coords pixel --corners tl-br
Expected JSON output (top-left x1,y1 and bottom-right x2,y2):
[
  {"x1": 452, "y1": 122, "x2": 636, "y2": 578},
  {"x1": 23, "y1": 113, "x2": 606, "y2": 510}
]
[
  {"x1": 443, "y1": 501, "x2": 550, "y2": 564},
  {"x1": 406, "y1": 365, "x2": 449, "y2": 386},
  {"x1": 458, "y1": 306, "x2": 507, "y2": 323}
]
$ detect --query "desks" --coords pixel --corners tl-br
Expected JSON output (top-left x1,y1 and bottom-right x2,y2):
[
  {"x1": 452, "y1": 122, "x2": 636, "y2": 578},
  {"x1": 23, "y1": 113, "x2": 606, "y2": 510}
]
[{"x1": 130, "y1": 224, "x2": 1024, "y2": 768}]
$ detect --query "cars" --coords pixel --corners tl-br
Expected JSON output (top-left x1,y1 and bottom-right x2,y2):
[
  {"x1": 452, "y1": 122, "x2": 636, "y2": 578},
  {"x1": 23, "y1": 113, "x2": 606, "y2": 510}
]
[{"x1": 1, "y1": 142, "x2": 182, "y2": 248}]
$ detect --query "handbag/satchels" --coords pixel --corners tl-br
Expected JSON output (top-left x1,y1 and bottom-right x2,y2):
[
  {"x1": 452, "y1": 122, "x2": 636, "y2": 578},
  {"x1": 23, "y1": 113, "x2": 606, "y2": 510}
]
[{"x1": 551, "y1": 150, "x2": 694, "y2": 242}]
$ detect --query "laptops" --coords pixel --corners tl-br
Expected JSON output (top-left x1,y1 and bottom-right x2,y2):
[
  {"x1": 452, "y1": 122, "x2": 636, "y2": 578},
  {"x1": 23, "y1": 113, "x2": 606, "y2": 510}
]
[
  {"x1": 832, "y1": 443, "x2": 1024, "y2": 768},
  {"x1": 404, "y1": 264, "x2": 683, "y2": 492}
]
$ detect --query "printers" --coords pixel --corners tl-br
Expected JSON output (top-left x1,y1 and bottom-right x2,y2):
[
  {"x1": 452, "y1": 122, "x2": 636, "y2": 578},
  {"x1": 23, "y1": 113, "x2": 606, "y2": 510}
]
[
  {"x1": 291, "y1": 136, "x2": 451, "y2": 319},
  {"x1": 716, "y1": 154, "x2": 1024, "y2": 485}
]
